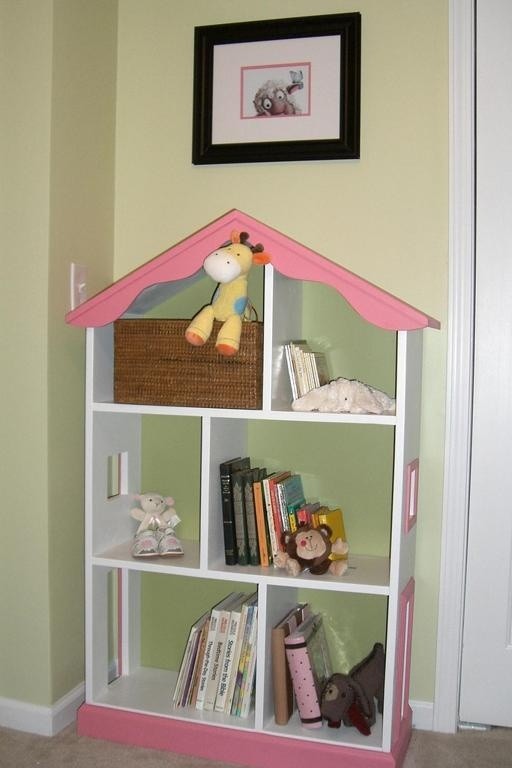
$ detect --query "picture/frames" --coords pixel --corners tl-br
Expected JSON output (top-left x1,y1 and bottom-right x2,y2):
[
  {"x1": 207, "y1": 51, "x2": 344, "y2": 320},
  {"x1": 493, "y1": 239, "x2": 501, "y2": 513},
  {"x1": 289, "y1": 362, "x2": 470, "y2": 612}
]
[{"x1": 191, "y1": 10, "x2": 364, "y2": 165}]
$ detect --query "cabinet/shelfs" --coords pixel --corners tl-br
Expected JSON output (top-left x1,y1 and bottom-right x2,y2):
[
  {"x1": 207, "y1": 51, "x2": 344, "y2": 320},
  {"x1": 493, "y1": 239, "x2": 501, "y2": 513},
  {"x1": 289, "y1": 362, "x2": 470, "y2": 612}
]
[{"x1": 66, "y1": 206, "x2": 443, "y2": 767}]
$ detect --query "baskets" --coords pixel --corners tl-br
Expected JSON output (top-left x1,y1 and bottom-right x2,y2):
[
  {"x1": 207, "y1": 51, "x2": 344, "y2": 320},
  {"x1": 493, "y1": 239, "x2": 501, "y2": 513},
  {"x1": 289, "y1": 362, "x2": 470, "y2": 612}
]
[{"x1": 113, "y1": 318, "x2": 264, "y2": 410}]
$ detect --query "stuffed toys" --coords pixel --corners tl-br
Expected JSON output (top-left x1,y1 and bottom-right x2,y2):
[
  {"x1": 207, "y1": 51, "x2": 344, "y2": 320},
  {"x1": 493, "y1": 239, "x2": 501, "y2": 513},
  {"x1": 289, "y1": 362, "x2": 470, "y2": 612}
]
[
  {"x1": 290, "y1": 378, "x2": 393, "y2": 414},
  {"x1": 184, "y1": 229, "x2": 272, "y2": 357},
  {"x1": 129, "y1": 492, "x2": 177, "y2": 536},
  {"x1": 320, "y1": 642, "x2": 384, "y2": 735},
  {"x1": 273, "y1": 522, "x2": 350, "y2": 575}
]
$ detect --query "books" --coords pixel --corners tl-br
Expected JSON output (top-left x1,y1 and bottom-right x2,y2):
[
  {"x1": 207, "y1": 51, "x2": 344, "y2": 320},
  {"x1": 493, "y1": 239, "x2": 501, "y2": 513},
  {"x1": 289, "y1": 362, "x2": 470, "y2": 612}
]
[
  {"x1": 283, "y1": 339, "x2": 329, "y2": 400},
  {"x1": 237, "y1": 601, "x2": 256, "y2": 718},
  {"x1": 297, "y1": 502, "x2": 318, "y2": 525},
  {"x1": 201, "y1": 592, "x2": 247, "y2": 713},
  {"x1": 310, "y1": 506, "x2": 328, "y2": 530},
  {"x1": 243, "y1": 468, "x2": 266, "y2": 565},
  {"x1": 287, "y1": 499, "x2": 304, "y2": 533},
  {"x1": 317, "y1": 509, "x2": 349, "y2": 561},
  {"x1": 231, "y1": 598, "x2": 257, "y2": 716},
  {"x1": 241, "y1": 606, "x2": 256, "y2": 715},
  {"x1": 231, "y1": 468, "x2": 258, "y2": 566},
  {"x1": 285, "y1": 612, "x2": 335, "y2": 728},
  {"x1": 219, "y1": 455, "x2": 249, "y2": 568},
  {"x1": 224, "y1": 593, "x2": 257, "y2": 717},
  {"x1": 262, "y1": 471, "x2": 292, "y2": 566},
  {"x1": 272, "y1": 602, "x2": 307, "y2": 726},
  {"x1": 193, "y1": 591, "x2": 248, "y2": 714},
  {"x1": 172, "y1": 609, "x2": 209, "y2": 710},
  {"x1": 269, "y1": 472, "x2": 288, "y2": 552},
  {"x1": 252, "y1": 473, "x2": 279, "y2": 566},
  {"x1": 274, "y1": 475, "x2": 305, "y2": 537},
  {"x1": 214, "y1": 592, "x2": 256, "y2": 713}
]
